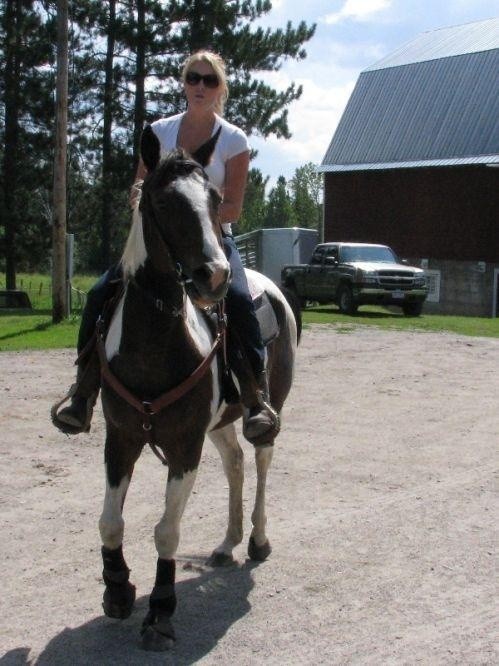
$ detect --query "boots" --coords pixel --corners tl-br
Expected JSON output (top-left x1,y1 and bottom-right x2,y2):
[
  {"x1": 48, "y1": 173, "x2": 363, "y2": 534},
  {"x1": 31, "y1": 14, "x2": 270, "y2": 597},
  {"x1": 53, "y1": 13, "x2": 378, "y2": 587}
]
[
  {"x1": 55, "y1": 366, "x2": 100, "y2": 432},
  {"x1": 242, "y1": 371, "x2": 274, "y2": 449}
]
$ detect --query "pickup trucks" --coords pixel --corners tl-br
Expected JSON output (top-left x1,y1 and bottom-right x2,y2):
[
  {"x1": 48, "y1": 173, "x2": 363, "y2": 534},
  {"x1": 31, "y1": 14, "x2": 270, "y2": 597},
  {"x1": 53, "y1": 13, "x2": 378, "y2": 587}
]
[{"x1": 276, "y1": 238, "x2": 430, "y2": 318}]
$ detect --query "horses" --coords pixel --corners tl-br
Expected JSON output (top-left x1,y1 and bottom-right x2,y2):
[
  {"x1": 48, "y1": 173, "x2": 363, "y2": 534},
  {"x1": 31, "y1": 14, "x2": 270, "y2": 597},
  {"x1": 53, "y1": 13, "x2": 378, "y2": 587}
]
[{"x1": 97, "y1": 123, "x2": 302, "y2": 652}]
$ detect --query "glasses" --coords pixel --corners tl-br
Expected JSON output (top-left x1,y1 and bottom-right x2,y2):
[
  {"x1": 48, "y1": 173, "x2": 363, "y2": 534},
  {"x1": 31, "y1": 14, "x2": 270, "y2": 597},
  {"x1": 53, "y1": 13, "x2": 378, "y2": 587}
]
[{"x1": 185, "y1": 71, "x2": 219, "y2": 88}]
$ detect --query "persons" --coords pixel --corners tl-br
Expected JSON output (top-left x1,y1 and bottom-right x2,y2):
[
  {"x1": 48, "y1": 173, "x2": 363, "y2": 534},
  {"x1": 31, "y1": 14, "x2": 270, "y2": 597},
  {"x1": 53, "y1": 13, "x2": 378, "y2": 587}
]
[{"x1": 54, "y1": 46, "x2": 277, "y2": 438}]
